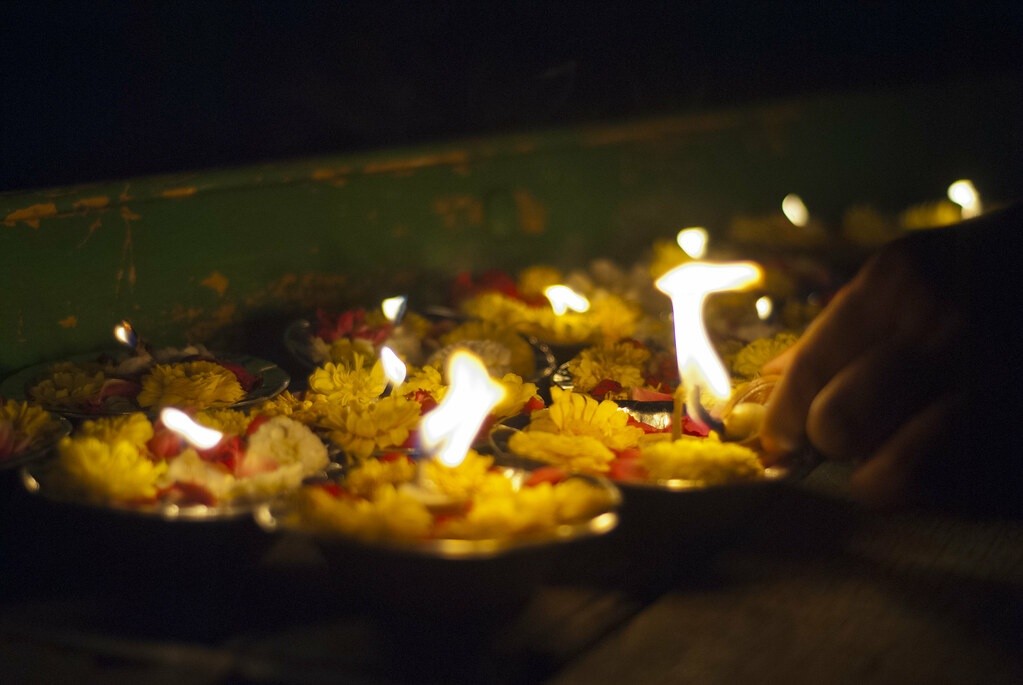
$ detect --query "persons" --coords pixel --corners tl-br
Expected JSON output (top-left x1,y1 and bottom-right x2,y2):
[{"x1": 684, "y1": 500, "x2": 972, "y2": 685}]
[{"x1": 747, "y1": 202, "x2": 1023, "y2": 507}]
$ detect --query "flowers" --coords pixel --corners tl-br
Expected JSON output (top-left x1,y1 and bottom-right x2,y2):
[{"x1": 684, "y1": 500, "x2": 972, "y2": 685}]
[{"x1": 0, "y1": 202, "x2": 991, "y2": 647}]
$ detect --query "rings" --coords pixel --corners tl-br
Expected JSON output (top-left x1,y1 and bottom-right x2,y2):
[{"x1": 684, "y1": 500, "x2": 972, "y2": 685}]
[{"x1": 720, "y1": 375, "x2": 779, "y2": 448}]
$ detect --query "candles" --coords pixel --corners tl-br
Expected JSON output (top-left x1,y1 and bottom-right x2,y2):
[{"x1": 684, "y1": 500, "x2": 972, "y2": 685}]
[{"x1": 652, "y1": 261, "x2": 793, "y2": 468}]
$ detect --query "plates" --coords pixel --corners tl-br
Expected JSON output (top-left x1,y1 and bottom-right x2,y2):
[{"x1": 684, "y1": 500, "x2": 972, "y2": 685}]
[
  {"x1": 0, "y1": 348, "x2": 291, "y2": 416},
  {"x1": 282, "y1": 303, "x2": 689, "y2": 423},
  {"x1": 0, "y1": 412, "x2": 795, "y2": 576}
]
